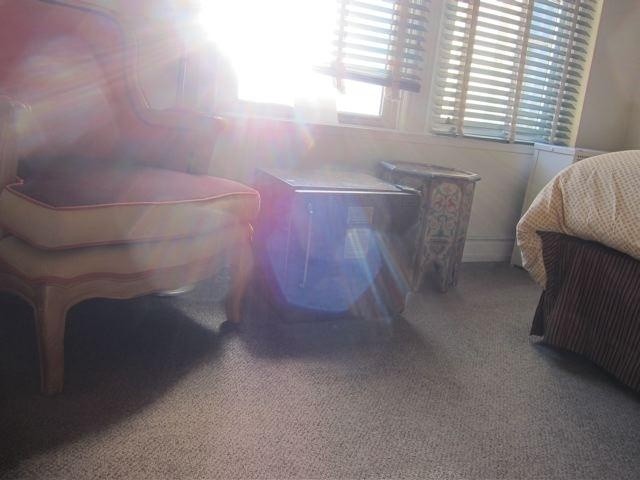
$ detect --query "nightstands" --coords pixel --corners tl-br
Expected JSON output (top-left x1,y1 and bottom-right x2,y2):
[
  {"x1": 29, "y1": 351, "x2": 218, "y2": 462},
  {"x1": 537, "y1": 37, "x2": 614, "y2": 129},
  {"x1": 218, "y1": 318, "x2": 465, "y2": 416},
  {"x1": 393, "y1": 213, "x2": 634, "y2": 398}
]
[{"x1": 510, "y1": 142, "x2": 610, "y2": 269}]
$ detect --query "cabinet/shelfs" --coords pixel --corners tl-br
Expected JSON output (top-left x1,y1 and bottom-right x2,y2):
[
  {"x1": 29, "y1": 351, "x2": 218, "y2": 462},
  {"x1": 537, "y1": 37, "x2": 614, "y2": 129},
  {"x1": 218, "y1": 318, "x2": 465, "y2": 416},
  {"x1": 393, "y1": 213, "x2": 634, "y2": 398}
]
[{"x1": 255, "y1": 164, "x2": 422, "y2": 321}]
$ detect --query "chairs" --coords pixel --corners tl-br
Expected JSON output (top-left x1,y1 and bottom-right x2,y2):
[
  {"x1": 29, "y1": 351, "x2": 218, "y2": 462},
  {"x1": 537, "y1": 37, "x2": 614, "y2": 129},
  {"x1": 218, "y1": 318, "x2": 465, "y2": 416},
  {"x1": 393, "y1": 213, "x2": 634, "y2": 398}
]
[{"x1": 0, "y1": 0, "x2": 294, "y2": 397}]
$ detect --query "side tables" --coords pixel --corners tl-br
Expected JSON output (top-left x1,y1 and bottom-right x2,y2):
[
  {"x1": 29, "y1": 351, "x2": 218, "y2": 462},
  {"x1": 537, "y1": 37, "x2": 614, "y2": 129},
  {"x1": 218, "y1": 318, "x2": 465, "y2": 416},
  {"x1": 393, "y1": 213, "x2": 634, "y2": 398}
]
[{"x1": 376, "y1": 159, "x2": 482, "y2": 293}]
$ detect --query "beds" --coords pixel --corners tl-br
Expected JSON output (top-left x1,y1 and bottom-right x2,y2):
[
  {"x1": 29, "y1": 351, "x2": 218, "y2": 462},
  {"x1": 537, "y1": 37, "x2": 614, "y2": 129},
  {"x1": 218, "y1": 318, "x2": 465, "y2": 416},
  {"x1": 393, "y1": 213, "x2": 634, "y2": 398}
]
[{"x1": 514, "y1": 151, "x2": 640, "y2": 396}]
